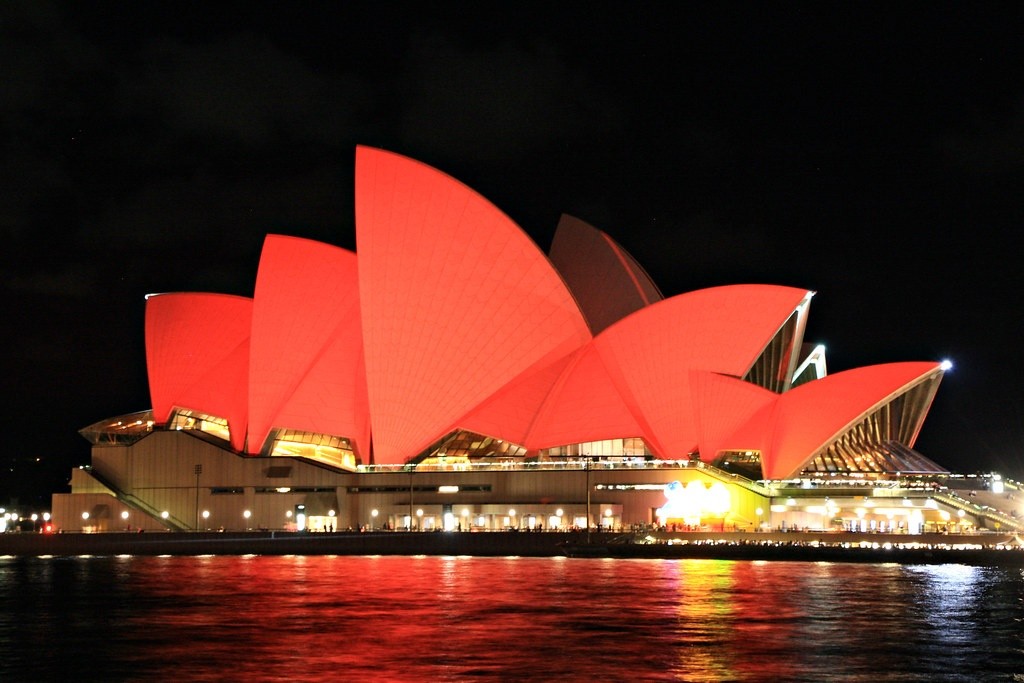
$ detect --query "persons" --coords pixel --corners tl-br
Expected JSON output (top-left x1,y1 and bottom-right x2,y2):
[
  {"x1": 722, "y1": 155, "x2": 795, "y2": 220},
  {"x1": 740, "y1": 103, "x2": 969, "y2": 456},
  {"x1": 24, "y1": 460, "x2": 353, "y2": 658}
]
[{"x1": 383, "y1": 522, "x2": 386, "y2": 530}]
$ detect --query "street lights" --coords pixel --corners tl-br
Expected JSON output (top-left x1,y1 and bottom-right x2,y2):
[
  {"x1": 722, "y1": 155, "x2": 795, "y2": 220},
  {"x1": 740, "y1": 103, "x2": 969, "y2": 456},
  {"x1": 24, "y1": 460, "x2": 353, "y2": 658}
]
[
  {"x1": 556, "y1": 508, "x2": 564, "y2": 533},
  {"x1": 371, "y1": 509, "x2": 379, "y2": 531},
  {"x1": 508, "y1": 508, "x2": 516, "y2": 529},
  {"x1": 82, "y1": 512, "x2": 90, "y2": 534},
  {"x1": 583, "y1": 452, "x2": 594, "y2": 544},
  {"x1": 461, "y1": 508, "x2": 469, "y2": 532},
  {"x1": 161, "y1": 511, "x2": 169, "y2": 531},
  {"x1": 194, "y1": 463, "x2": 203, "y2": 532},
  {"x1": 202, "y1": 510, "x2": 210, "y2": 532},
  {"x1": 121, "y1": 511, "x2": 129, "y2": 532},
  {"x1": 243, "y1": 510, "x2": 252, "y2": 532},
  {"x1": 11, "y1": 513, "x2": 19, "y2": 530},
  {"x1": 286, "y1": 510, "x2": 293, "y2": 532},
  {"x1": 43, "y1": 512, "x2": 50, "y2": 534},
  {"x1": 416, "y1": 507, "x2": 424, "y2": 532},
  {"x1": 31, "y1": 514, "x2": 38, "y2": 534},
  {"x1": 407, "y1": 457, "x2": 416, "y2": 532},
  {"x1": 328, "y1": 509, "x2": 336, "y2": 532}
]
